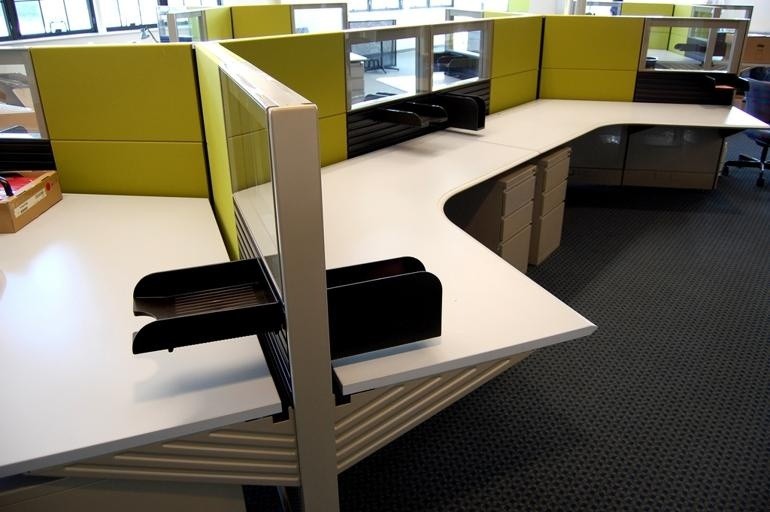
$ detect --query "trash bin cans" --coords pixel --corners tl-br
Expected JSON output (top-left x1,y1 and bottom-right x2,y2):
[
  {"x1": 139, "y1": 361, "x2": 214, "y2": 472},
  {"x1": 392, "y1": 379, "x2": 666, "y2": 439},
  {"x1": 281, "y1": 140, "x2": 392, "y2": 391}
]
[{"x1": 737, "y1": 65, "x2": 770, "y2": 140}]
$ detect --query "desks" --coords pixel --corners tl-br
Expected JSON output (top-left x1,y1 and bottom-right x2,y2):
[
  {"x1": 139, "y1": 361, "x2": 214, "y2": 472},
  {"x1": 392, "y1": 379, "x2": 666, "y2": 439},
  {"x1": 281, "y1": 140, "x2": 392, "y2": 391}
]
[
  {"x1": 647, "y1": 48, "x2": 700, "y2": 66},
  {"x1": 349, "y1": 51, "x2": 369, "y2": 97},
  {"x1": 0, "y1": 192, "x2": 286, "y2": 484},
  {"x1": 376, "y1": 69, "x2": 470, "y2": 97},
  {"x1": 236, "y1": 97, "x2": 770, "y2": 405}
]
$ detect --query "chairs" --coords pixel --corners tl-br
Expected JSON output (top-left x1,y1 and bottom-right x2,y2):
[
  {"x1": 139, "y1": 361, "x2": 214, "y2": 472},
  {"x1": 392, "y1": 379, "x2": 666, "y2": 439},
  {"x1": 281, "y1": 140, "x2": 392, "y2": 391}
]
[{"x1": 719, "y1": 66, "x2": 770, "y2": 194}]
